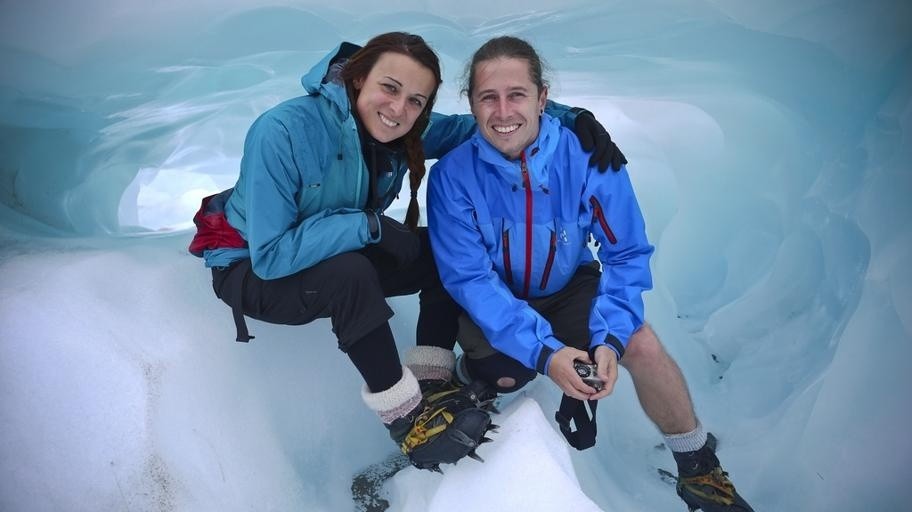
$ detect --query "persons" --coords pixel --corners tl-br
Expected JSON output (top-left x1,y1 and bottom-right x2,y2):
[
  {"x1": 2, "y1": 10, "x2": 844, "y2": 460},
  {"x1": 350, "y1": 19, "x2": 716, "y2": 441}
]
[
  {"x1": 198, "y1": 28, "x2": 627, "y2": 470},
  {"x1": 425, "y1": 33, "x2": 757, "y2": 511}
]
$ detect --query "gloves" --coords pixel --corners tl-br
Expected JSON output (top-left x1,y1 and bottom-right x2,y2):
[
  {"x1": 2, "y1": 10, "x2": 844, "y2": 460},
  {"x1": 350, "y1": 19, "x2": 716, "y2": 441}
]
[
  {"x1": 576, "y1": 112, "x2": 627, "y2": 173},
  {"x1": 371, "y1": 215, "x2": 421, "y2": 266}
]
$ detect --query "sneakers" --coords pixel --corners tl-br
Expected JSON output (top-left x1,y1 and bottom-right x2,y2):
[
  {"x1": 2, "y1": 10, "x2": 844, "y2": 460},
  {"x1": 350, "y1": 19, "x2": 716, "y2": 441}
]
[{"x1": 383, "y1": 379, "x2": 490, "y2": 467}]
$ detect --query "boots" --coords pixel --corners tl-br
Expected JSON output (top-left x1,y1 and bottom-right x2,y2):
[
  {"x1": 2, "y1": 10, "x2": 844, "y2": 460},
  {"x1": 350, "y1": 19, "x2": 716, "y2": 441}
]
[{"x1": 672, "y1": 432, "x2": 754, "y2": 512}]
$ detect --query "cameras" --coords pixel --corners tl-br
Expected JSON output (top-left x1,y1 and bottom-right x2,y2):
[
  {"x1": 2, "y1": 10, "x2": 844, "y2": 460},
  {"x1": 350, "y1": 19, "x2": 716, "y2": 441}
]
[{"x1": 571, "y1": 364, "x2": 604, "y2": 386}]
